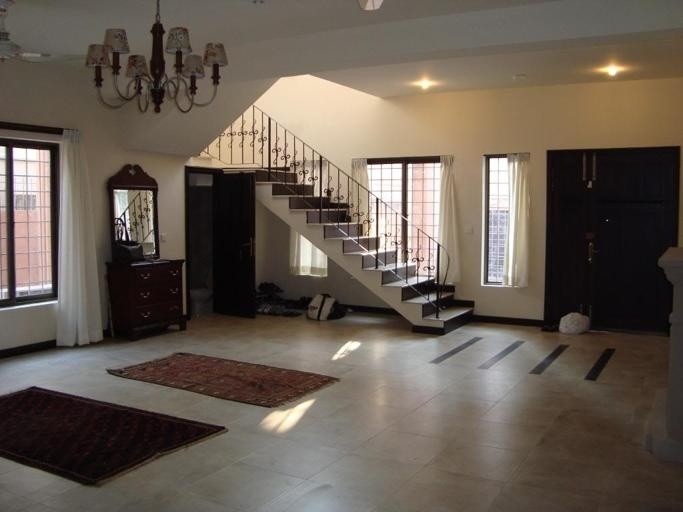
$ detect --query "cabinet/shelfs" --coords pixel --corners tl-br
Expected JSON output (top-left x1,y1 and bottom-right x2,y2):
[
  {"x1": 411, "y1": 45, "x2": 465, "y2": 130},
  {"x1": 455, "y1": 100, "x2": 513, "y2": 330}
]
[{"x1": 106, "y1": 258, "x2": 192, "y2": 341}]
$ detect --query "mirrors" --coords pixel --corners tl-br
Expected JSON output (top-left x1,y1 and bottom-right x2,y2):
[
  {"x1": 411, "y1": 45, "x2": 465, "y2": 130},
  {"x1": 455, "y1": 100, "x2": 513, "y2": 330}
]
[{"x1": 108, "y1": 164, "x2": 159, "y2": 258}]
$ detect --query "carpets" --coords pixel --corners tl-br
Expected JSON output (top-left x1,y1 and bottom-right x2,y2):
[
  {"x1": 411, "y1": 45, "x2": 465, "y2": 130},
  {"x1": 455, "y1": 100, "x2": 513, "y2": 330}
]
[
  {"x1": 106, "y1": 352, "x2": 339, "y2": 410},
  {"x1": 1, "y1": 386, "x2": 228, "y2": 488}
]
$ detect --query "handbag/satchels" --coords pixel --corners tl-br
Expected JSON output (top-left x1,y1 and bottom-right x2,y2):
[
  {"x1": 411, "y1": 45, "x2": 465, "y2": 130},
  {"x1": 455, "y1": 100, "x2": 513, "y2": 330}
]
[{"x1": 307, "y1": 294, "x2": 345, "y2": 320}]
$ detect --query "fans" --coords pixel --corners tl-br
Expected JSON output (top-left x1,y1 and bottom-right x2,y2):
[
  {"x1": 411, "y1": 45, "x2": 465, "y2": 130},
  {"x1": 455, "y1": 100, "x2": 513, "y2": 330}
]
[{"x1": 1, "y1": 1, "x2": 87, "y2": 66}]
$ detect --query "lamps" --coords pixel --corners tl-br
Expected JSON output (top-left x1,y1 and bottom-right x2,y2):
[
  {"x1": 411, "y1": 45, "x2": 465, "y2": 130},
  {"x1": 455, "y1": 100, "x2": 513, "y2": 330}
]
[{"x1": 85, "y1": 0, "x2": 229, "y2": 115}]
